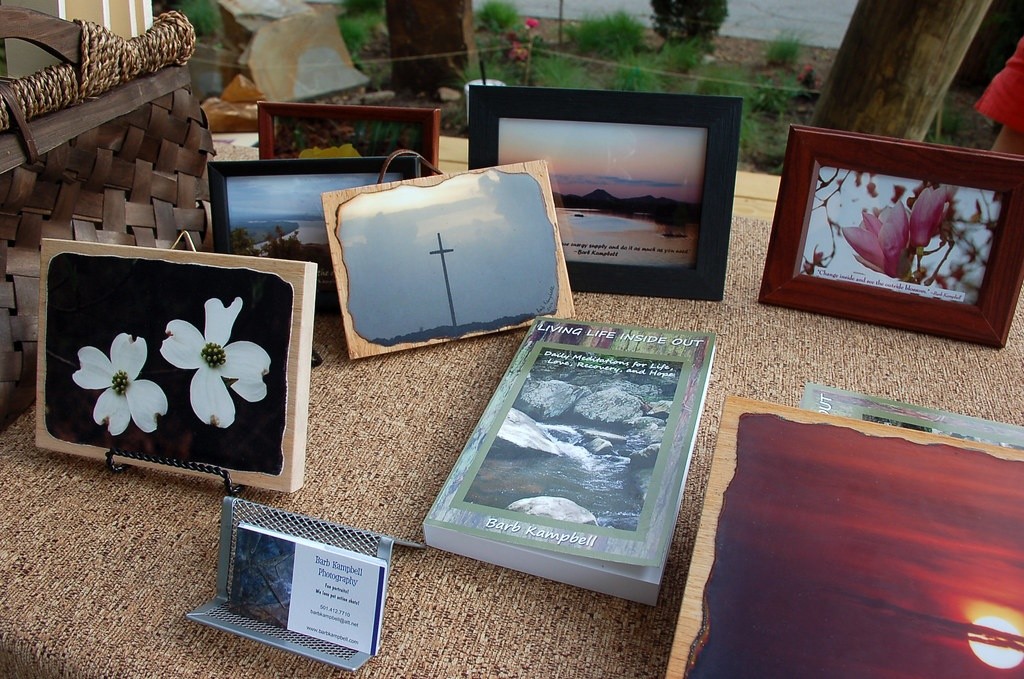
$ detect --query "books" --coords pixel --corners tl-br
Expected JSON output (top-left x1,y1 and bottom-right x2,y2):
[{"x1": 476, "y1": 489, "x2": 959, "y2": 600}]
[{"x1": 423, "y1": 315, "x2": 715, "y2": 607}]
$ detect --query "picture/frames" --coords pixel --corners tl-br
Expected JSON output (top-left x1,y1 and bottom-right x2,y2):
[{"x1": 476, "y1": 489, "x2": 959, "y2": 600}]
[
  {"x1": 34, "y1": 238, "x2": 319, "y2": 493},
  {"x1": 757, "y1": 124, "x2": 1024, "y2": 347},
  {"x1": 206, "y1": 157, "x2": 423, "y2": 318},
  {"x1": 661, "y1": 398, "x2": 1024, "y2": 678},
  {"x1": 465, "y1": 81, "x2": 744, "y2": 301},
  {"x1": 256, "y1": 98, "x2": 441, "y2": 186},
  {"x1": 322, "y1": 157, "x2": 577, "y2": 362}
]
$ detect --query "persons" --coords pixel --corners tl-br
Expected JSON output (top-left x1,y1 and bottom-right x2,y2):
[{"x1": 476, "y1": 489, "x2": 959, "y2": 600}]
[{"x1": 973, "y1": 37, "x2": 1024, "y2": 155}]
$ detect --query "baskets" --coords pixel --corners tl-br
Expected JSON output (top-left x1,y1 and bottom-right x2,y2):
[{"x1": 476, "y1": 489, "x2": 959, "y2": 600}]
[{"x1": 0, "y1": 6, "x2": 216, "y2": 434}]
